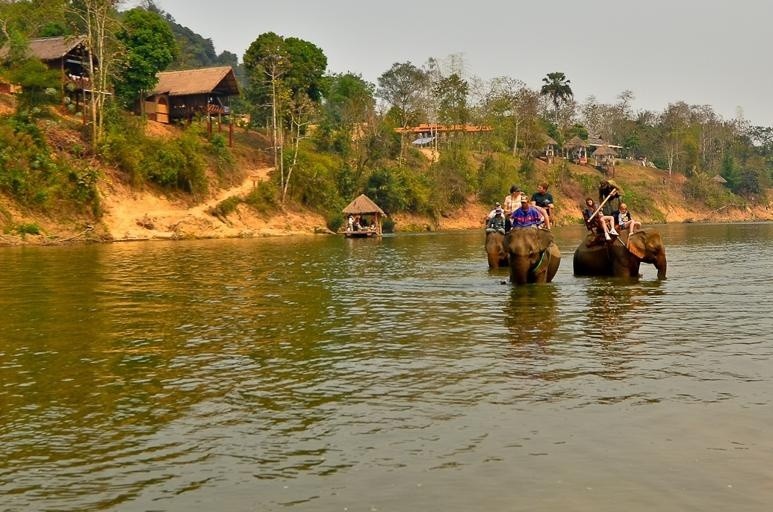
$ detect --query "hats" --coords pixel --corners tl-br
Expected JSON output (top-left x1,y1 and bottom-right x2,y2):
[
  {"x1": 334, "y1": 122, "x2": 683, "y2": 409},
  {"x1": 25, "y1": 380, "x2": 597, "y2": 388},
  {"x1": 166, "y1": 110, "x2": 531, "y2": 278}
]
[
  {"x1": 510, "y1": 186, "x2": 522, "y2": 193},
  {"x1": 493, "y1": 209, "x2": 503, "y2": 214},
  {"x1": 521, "y1": 196, "x2": 529, "y2": 203},
  {"x1": 600, "y1": 181, "x2": 608, "y2": 190}
]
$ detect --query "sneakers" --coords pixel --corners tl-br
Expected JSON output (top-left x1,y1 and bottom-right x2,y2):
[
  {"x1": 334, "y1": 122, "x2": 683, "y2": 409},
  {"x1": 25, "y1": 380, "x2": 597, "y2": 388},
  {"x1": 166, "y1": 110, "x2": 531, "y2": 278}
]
[
  {"x1": 604, "y1": 233, "x2": 612, "y2": 241},
  {"x1": 608, "y1": 230, "x2": 620, "y2": 236}
]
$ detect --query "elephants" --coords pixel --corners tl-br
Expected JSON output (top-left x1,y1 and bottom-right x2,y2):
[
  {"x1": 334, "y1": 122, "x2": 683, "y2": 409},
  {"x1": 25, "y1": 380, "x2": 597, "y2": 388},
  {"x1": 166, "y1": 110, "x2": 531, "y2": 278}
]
[
  {"x1": 573, "y1": 227, "x2": 668, "y2": 281},
  {"x1": 484, "y1": 230, "x2": 509, "y2": 269},
  {"x1": 503, "y1": 226, "x2": 562, "y2": 288}
]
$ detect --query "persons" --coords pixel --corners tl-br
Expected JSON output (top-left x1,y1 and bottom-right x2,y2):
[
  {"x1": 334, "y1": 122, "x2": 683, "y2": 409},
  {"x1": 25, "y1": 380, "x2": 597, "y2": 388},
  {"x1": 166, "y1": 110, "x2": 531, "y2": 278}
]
[
  {"x1": 348, "y1": 212, "x2": 376, "y2": 232},
  {"x1": 618, "y1": 203, "x2": 642, "y2": 237},
  {"x1": 486, "y1": 183, "x2": 556, "y2": 232},
  {"x1": 598, "y1": 178, "x2": 622, "y2": 229},
  {"x1": 583, "y1": 197, "x2": 620, "y2": 242}
]
